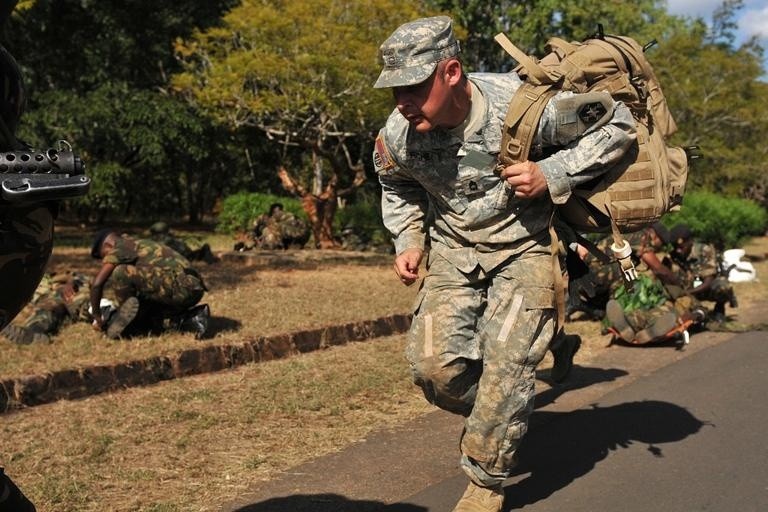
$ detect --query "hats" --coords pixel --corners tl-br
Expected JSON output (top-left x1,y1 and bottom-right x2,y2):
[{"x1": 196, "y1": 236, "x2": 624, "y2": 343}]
[
  {"x1": 650, "y1": 223, "x2": 673, "y2": 246},
  {"x1": 372, "y1": 15, "x2": 462, "y2": 89},
  {"x1": 670, "y1": 224, "x2": 691, "y2": 246},
  {"x1": 92, "y1": 227, "x2": 113, "y2": 259}
]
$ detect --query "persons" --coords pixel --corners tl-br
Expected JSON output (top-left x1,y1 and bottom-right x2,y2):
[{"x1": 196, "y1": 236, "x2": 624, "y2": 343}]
[
  {"x1": 90, "y1": 229, "x2": 212, "y2": 343},
  {"x1": 0, "y1": 1, "x2": 58, "y2": 512},
  {"x1": 565, "y1": 220, "x2": 738, "y2": 345},
  {"x1": 235, "y1": 205, "x2": 309, "y2": 251},
  {"x1": 146, "y1": 222, "x2": 213, "y2": 266},
  {"x1": 373, "y1": 16, "x2": 639, "y2": 512},
  {"x1": 1, "y1": 269, "x2": 139, "y2": 343}
]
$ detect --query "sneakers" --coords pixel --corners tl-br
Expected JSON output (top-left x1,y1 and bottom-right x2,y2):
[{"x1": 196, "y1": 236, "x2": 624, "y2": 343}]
[
  {"x1": 550, "y1": 334, "x2": 581, "y2": 384},
  {"x1": 453, "y1": 481, "x2": 505, "y2": 511}
]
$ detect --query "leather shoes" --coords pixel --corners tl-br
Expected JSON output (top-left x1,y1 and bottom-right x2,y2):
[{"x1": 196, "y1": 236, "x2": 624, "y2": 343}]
[
  {"x1": 712, "y1": 304, "x2": 725, "y2": 320},
  {"x1": 191, "y1": 303, "x2": 210, "y2": 339},
  {"x1": 105, "y1": 296, "x2": 141, "y2": 339},
  {"x1": 635, "y1": 313, "x2": 677, "y2": 345},
  {"x1": 564, "y1": 293, "x2": 583, "y2": 323},
  {"x1": 3, "y1": 324, "x2": 51, "y2": 346},
  {"x1": 606, "y1": 299, "x2": 635, "y2": 343}
]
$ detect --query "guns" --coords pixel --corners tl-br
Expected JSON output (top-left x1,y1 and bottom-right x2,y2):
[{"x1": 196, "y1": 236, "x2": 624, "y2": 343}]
[{"x1": 0, "y1": 139, "x2": 90, "y2": 205}]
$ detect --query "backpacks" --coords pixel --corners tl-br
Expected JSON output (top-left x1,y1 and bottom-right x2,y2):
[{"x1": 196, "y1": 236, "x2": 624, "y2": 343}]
[{"x1": 493, "y1": 22, "x2": 690, "y2": 233}]
